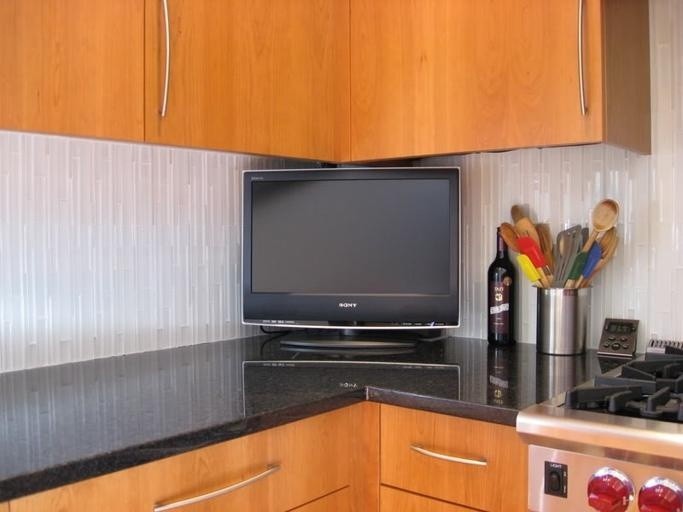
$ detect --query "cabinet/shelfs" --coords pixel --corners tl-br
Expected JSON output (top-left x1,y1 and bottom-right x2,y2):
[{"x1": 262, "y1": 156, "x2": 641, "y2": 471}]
[
  {"x1": 380, "y1": 402, "x2": 527, "y2": 510},
  {"x1": 352, "y1": 0, "x2": 651, "y2": 165},
  {"x1": 2, "y1": 1, "x2": 146, "y2": 144},
  {"x1": 146, "y1": 0, "x2": 351, "y2": 164},
  {"x1": 377, "y1": 485, "x2": 478, "y2": 512},
  {"x1": 10, "y1": 402, "x2": 353, "y2": 510}
]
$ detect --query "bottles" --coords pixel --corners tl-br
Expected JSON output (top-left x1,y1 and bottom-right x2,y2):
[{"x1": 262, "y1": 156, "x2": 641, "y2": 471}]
[
  {"x1": 488, "y1": 226, "x2": 515, "y2": 348},
  {"x1": 487, "y1": 346, "x2": 513, "y2": 409}
]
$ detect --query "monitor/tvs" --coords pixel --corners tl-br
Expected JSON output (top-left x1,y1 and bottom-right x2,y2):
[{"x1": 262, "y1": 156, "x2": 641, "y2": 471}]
[
  {"x1": 238, "y1": 167, "x2": 463, "y2": 351},
  {"x1": 241, "y1": 360, "x2": 461, "y2": 417}
]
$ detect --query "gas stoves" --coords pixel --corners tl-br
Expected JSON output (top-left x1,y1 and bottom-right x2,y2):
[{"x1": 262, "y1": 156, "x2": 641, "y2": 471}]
[{"x1": 514, "y1": 338, "x2": 682, "y2": 512}]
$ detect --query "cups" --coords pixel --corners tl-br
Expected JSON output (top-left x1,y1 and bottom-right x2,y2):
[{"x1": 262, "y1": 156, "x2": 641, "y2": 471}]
[
  {"x1": 536, "y1": 351, "x2": 587, "y2": 404},
  {"x1": 537, "y1": 282, "x2": 591, "y2": 356}
]
